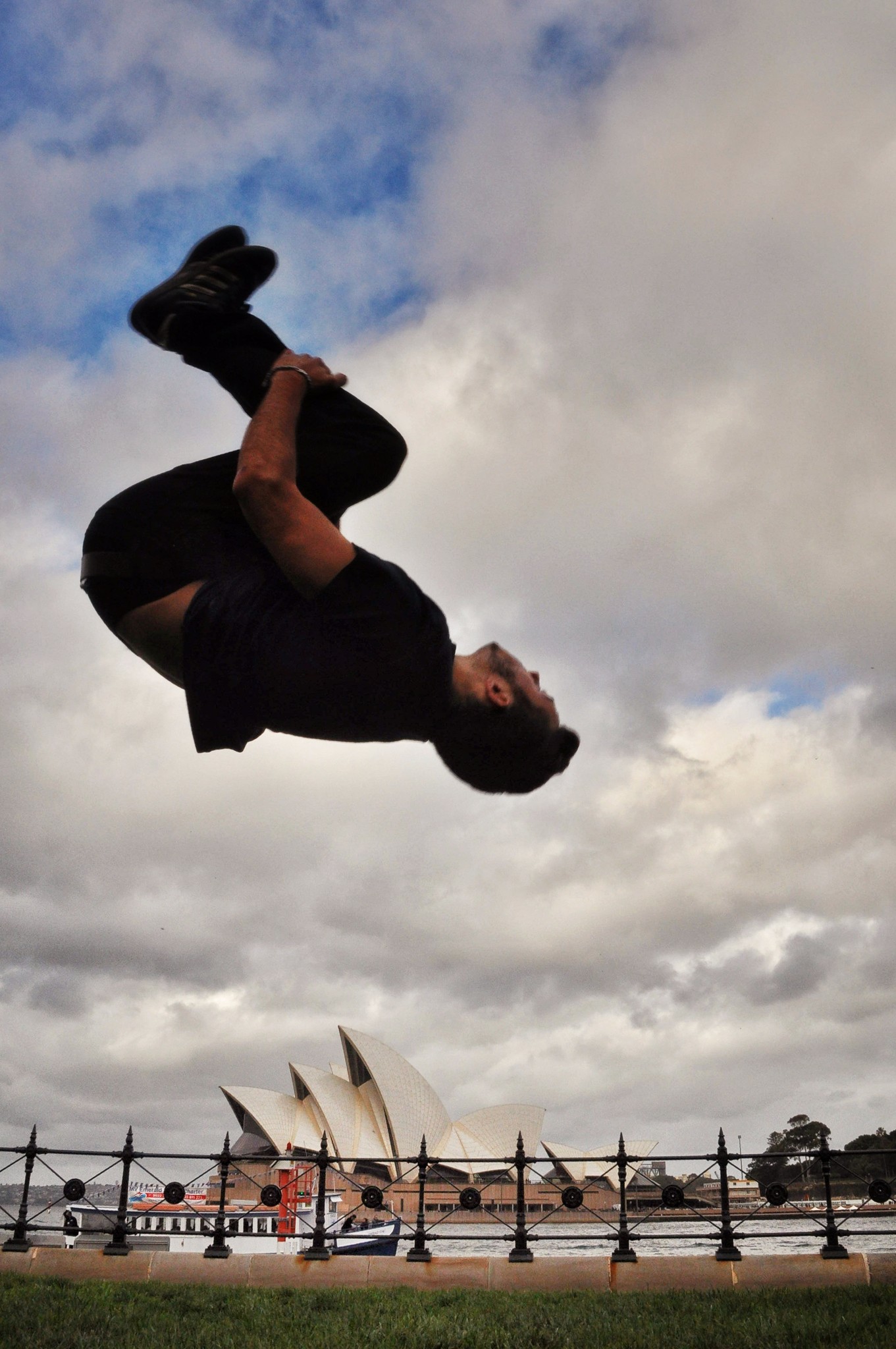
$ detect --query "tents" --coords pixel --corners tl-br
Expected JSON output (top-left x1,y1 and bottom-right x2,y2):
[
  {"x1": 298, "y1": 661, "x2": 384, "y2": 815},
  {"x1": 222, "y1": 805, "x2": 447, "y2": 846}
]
[{"x1": 809, "y1": 1205, "x2": 863, "y2": 1211}]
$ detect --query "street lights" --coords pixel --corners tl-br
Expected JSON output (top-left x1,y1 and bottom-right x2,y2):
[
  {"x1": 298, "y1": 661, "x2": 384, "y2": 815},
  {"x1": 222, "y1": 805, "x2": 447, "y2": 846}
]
[{"x1": 738, "y1": 1135, "x2": 743, "y2": 1180}]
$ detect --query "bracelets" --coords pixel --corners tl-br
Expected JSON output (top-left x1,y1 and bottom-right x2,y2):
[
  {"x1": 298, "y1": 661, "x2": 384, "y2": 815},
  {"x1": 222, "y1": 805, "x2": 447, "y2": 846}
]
[{"x1": 268, "y1": 365, "x2": 312, "y2": 390}]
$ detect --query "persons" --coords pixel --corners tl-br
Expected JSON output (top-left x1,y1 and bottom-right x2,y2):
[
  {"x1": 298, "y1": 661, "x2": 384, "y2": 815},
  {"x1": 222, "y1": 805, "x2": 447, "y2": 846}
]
[
  {"x1": 341, "y1": 1214, "x2": 356, "y2": 1234},
  {"x1": 372, "y1": 1215, "x2": 379, "y2": 1228},
  {"x1": 360, "y1": 1218, "x2": 369, "y2": 1231},
  {"x1": 83, "y1": 225, "x2": 580, "y2": 794}
]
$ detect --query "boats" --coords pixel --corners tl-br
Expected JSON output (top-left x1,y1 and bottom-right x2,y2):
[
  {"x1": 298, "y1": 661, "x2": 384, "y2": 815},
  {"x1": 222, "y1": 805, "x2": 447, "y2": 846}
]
[{"x1": 65, "y1": 1141, "x2": 401, "y2": 1256}]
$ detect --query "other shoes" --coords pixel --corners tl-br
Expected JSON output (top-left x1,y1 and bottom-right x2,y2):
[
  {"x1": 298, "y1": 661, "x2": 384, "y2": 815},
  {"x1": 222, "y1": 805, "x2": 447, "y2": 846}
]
[{"x1": 127, "y1": 226, "x2": 277, "y2": 354}]
